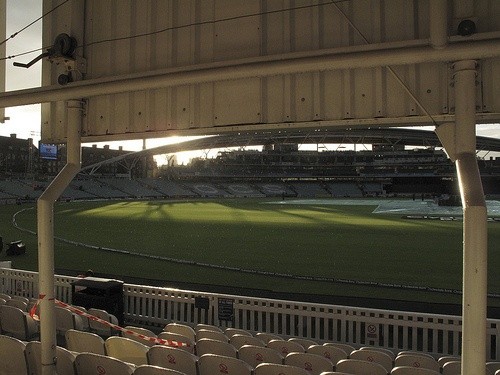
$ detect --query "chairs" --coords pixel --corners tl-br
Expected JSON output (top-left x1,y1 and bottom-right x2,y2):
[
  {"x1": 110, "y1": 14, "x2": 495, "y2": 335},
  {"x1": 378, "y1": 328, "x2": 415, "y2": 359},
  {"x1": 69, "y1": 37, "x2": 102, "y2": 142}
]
[{"x1": 0, "y1": 294, "x2": 500, "y2": 375}]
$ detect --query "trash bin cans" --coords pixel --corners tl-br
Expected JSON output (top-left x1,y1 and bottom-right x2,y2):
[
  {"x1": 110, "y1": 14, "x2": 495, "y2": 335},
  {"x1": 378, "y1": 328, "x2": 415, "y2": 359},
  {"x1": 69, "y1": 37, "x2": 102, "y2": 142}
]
[{"x1": 71, "y1": 277, "x2": 125, "y2": 327}]
